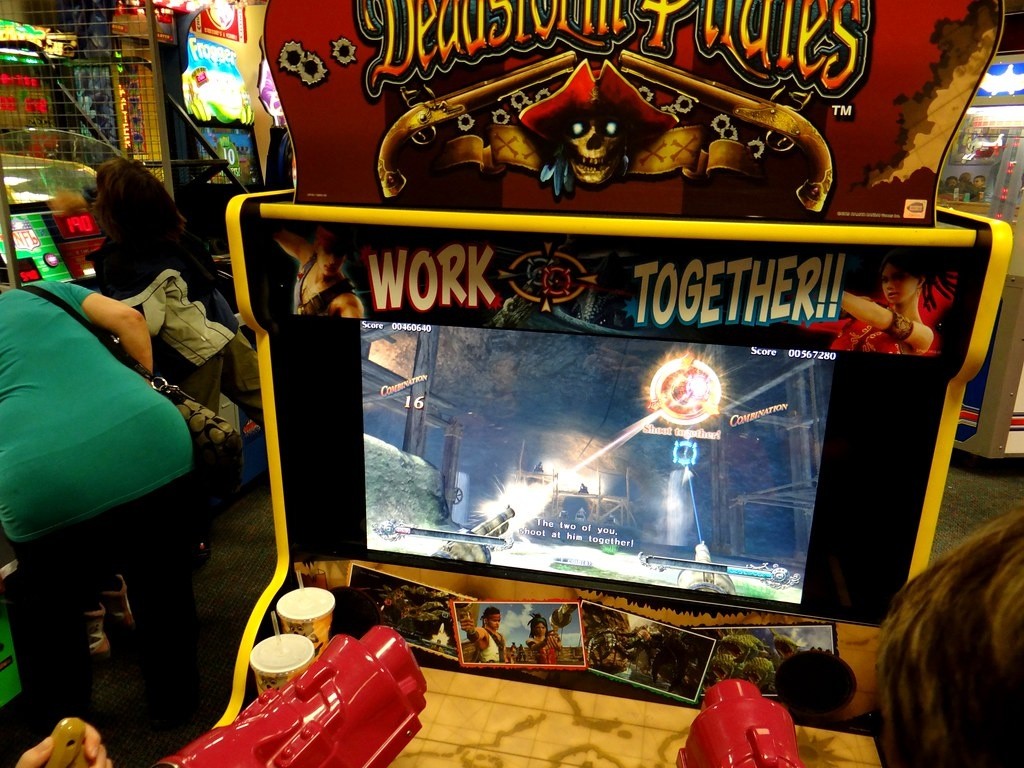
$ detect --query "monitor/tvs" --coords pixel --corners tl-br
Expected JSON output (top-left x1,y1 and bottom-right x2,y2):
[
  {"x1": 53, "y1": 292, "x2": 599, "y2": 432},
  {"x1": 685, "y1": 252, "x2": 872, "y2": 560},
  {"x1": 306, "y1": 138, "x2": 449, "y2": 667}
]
[{"x1": 361, "y1": 320, "x2": 834, "y2": 605}]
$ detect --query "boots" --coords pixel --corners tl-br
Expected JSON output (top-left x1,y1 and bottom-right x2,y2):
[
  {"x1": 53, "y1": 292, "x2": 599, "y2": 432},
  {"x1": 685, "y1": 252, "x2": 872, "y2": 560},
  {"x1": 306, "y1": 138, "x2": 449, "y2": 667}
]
[
  {"x1": 83, "y1": 602, "x2": 111, "y2": 661},
  {"x1": 101, "y1": 574, "x2": 136, "y2": 631}
]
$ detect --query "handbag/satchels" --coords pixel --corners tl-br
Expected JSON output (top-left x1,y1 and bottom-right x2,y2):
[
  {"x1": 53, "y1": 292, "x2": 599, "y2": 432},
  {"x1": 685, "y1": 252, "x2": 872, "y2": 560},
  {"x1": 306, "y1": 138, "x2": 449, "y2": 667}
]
[{"x1": 150, "y1": 377, "x2": 243, "y2": 470}]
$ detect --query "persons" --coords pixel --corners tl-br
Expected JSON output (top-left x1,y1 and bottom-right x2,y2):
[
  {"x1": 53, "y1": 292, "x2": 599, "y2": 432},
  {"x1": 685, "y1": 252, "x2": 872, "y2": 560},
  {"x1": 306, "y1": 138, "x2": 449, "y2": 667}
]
[
  {"x1": 15, "y1": 717, "x2": 113, "y2": 768},
  {"x1": 874, "y1": 510, "x2": 1024, "y2": 766},
  {"x1": 70, "y1": 158, "x2": 279, "y2": 567},
  {"x1": 0, "y1": 280, "x2": 198, "y2": 731}
]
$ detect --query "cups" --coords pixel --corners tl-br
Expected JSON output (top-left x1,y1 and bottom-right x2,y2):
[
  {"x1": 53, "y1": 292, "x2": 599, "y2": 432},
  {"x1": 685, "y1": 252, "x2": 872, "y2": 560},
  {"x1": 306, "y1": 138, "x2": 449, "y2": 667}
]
[
  {"x1": 248, "y1": 634, "x2": 315, "y2": 696},
  {"x1": 276, "y1": 586, "x2": 336, "y2": 657}
]
